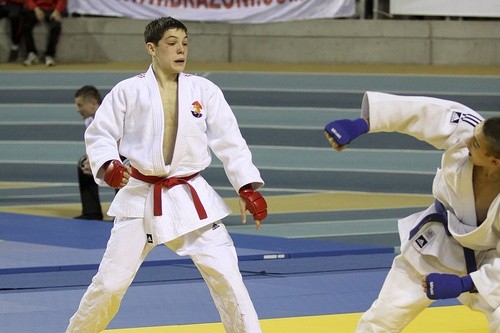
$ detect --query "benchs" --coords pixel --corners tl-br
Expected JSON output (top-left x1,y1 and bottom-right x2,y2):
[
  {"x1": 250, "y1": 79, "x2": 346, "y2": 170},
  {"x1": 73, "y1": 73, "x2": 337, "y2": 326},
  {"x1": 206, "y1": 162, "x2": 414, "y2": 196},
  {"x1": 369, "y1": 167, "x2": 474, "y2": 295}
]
[
  {"x1": 428, "y1": 20, "x2": 500, "y2": 67},
  {"x1": 1, "y1": 18, "x2": 230, "y2": 63},
  {"x1": 229, "y1": 20, "x2": 432, "y2": 64}
]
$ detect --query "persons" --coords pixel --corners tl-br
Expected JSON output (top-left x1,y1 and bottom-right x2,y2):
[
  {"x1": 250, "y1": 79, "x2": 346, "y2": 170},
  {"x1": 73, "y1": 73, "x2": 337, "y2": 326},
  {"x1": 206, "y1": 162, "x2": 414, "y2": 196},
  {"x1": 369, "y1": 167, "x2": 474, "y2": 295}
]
[
  {"x1": 73, "y1": 83, "x2": 127, "y2": 219},
  {"x1": 0, "y1": 0, "x2": 23, "y2": 63},
  {"x1": 66, "y1": 16, "x2": 268, "y2": 332},
  {"x1": 322, "y1": 91, "x2": 500, "y2": 333},
  {"x1": 20, "y1": 0, "x2": 66, "y2": 66}
]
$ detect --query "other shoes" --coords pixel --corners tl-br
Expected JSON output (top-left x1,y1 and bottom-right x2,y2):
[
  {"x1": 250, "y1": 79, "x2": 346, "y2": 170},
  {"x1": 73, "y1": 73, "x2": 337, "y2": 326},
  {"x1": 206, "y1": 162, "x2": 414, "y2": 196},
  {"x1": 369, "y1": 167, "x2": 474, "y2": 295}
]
[
  {"x1": 74, "y1": 214, "x2": 104, "y2": 220},
  {"x1": 45, "y1": 56, "x2": 56, "y2": 66},
  {"x1": 23, "y1": 53, "x2": 39, "y2": 66}
]
